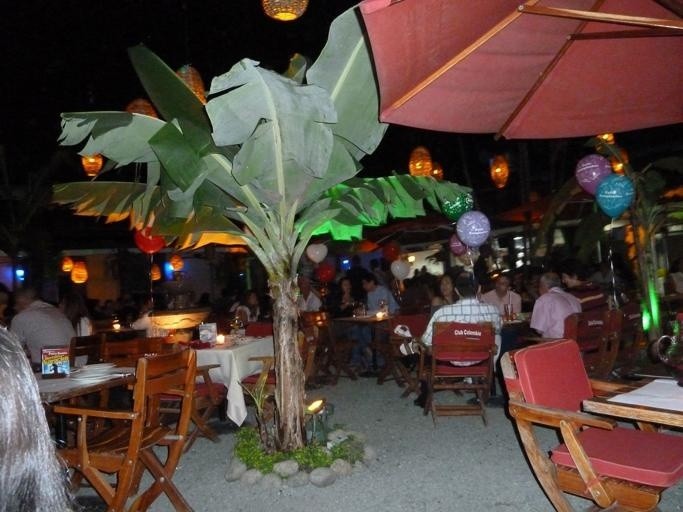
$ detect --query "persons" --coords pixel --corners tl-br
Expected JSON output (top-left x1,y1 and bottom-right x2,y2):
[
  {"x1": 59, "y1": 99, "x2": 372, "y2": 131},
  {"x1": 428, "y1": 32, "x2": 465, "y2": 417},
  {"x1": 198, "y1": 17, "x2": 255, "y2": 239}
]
[
  {"x1": 1, "y1": 325, "x2": 78, "y2": 512},
  {"x1": 496, "y1": 271, "x2": 583, "y2": 409},
  {"x1": 74, "y1": 289, "x2": 218, "y2": 334},
  {"x1": 334, "y1": 278, "x2": 357, "y2": 318},
  {"x1": 480, "y1": 273, "x2": 521, "y2": 319},
  {"x1": 350, "y1": 272, "x2": 400, "y2": 370},
  {"x1": 231, "y1": 291, "x2": 260, "y2": 336},
  {"x1": 9, "y1": 283, "x2": 76, "y2": 372},
  {"x1": 399, "y1": 276, "x2": 502, "y2": 411},
  {"x1": 562, "y1": 260, "x2": 608, "y2": 339},
  {"x1": 1, "y1": 284, "x2": 17, "y2": 328},
  {"x1": 298, "y1": 278, "x2": 322, "y2": 311},
  {"x1": 431, "y1": 275, "x2": 456, "y2": 320}
]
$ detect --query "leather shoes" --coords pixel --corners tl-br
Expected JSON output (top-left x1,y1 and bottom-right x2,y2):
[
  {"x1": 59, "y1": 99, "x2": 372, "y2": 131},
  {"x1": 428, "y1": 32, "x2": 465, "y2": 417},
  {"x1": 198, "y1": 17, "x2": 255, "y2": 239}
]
[
  {"x1": 357, "y1": 370, "x2": 372, "y2": 380},
  {"x1": 372, "y1": 370, "x2": 381, "y2": 379}
]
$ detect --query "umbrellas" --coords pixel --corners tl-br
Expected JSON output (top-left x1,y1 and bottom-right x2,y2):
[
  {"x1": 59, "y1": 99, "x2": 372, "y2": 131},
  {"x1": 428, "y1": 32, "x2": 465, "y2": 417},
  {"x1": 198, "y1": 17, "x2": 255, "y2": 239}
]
[{"x1": 358, "y1": 0, "x2": 683, "y2": 141}]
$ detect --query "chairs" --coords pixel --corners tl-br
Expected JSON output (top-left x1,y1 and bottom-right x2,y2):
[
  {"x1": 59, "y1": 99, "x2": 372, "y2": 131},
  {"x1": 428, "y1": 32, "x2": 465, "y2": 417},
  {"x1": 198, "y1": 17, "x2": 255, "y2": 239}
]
[
  {"x1": 562, "y1": 308, "x2": 622, "y2": 381},
  {"x1": 298, "y1": 310, "x2": 358, "y2": 384},
  {"x1": 501, "y1": 338, "x2": 683, "y2": 511},
  {"x1": 52, "y1": 348, "x2": 199, "y2": 511},
  {"x1": 416, "y1": 322, "x2": 498, "y2": 426},
  {"x1": 376, "y1": 313, "x2": 429, "y2": 386},
  {"x1": 240, "y1": 322, "x2": 276, "y2": 395},
  {"x1": 143, "y1": 346, "x2": 227, "y2": 454},
  {"x1": 68, "y1": 335, "x2": 102, "y2": 368},
  {"x1": 610, "y1": 300, "x2": 643, "y2": 373}
]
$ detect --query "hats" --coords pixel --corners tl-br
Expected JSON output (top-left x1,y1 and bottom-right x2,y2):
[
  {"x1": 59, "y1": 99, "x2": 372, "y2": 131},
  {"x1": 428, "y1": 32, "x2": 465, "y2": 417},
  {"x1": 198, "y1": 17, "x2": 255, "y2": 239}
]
[
  {"x1": 553, "y1": 262, "x2": 591, "y2": 285},
  {"x1": 358, "y1": 272, "x2": 377, "y2": 281}
]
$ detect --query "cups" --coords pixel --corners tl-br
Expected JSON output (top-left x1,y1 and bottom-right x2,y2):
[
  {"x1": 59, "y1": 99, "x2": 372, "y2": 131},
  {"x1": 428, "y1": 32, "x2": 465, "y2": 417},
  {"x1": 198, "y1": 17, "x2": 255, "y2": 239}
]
[{"x1": 504, "y1": 303, "x2": 513, "y2": 321}]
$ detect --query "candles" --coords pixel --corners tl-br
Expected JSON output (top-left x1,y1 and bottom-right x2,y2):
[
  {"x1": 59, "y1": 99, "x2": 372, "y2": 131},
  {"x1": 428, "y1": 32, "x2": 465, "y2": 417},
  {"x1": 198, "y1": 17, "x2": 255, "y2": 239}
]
[
  {"x1": 216, "y1": 334, "x2": 225, "y2": 346},
  {"x1": 112, "y1": 319, "x2": 121, "y2": 331}
]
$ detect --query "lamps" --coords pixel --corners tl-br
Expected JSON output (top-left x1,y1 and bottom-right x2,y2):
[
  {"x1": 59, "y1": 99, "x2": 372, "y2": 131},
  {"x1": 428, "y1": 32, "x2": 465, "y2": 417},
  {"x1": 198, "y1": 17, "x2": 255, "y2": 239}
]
[
  {"x1": 409, "y1": 145, "x2": 432, "y2": 177},
  {"x1": 61, "y1": 256, "x2": 74, "y2": 272},
  {"x1": 171, "y1": 253, "x2": 184, "y2": 270},
  {"x1": 491, "y1": 152, "x2": 509, "y2": 189},
  {"x1": 82, "y1": 150, "x2": 102, "y2": 175},
  {"x1": 72, "y1": 257, "x2": 87, "y2": 283},
  {"x1": 150, "y1": 262, "x2": 160, "y2": 281}
]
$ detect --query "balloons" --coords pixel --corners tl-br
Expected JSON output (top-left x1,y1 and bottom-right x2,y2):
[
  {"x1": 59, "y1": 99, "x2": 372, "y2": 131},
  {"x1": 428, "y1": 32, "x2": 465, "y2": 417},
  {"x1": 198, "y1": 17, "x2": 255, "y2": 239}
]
[
  {"x1": 306, "y1": 244, "x2": 328, "y2": 263},
  {"x1": 450, "y1": 234, "x2": 468, "y2": 257},
  {"x1": 596, "y1": 175, "x2": 635, "y2": 219},
  {"x1": 316, "y1": 265, "x2": 336, "y2": 282},
  {"x1": 575, "y1": 155, "x2": 612, "y2": 196},
  {"x1": 383, "y1": 242, "x2": 400, "y2": 263},
  {"x1": 456, "y1": 211, "x2": 492, "y2": 250},
  {"x1": 340, "y1": 257, "x2": 355, "y2": 271},
  {"x1": 135, "y1": 227, "x2": 166, "y2": 254},
  {"x1": 390, "y1": 258, "x2": 410, "y2": 281}
]
